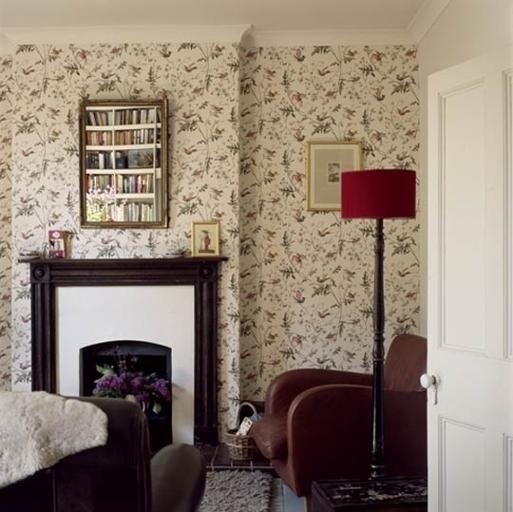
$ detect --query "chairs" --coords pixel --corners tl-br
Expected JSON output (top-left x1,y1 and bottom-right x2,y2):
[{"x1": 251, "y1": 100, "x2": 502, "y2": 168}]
[{"x1": 254, "y1": 334, "x2": 426, "y2": 511}]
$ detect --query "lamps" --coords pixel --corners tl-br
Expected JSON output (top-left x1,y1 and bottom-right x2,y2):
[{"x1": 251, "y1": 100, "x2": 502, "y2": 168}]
[{"x1": 339, "y1": 168, "x2": 417, "y2": 486}]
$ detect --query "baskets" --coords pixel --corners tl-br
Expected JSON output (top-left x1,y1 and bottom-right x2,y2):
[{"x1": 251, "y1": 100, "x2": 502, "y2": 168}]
[{"x1": 223, "y1": 401, "x2": 262, "y2": 461}]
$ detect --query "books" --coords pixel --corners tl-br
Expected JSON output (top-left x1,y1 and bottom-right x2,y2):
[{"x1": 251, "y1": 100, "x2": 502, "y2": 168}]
[{"x1": 85, "y1": 106, "x2": 162, "y2": 223}]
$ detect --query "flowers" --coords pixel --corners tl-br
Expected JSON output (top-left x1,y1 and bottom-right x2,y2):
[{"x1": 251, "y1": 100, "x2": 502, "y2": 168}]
[{"x1": 88, "y1": 348, "x2": 168, "y2": 417}]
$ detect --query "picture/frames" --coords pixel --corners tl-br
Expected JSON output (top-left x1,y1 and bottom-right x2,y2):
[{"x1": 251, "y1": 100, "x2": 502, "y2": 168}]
[
  {"x1": 307, "y1": 139, "x2": 362, "y2": 211},
  {"x1": 190, "y1": 222, "x2": 221, "y2": 257}
]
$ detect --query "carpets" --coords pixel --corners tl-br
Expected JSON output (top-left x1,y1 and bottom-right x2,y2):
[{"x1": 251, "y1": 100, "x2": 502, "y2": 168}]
[{"x1": 197, "y1": 469, "x2": 274, "y2": 512}]
